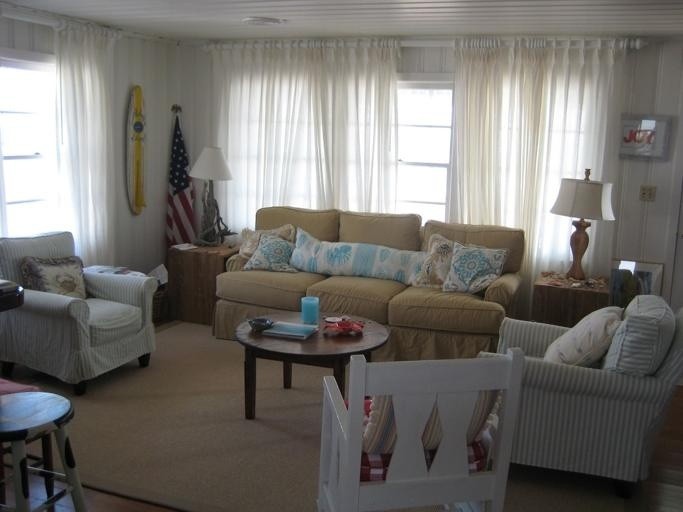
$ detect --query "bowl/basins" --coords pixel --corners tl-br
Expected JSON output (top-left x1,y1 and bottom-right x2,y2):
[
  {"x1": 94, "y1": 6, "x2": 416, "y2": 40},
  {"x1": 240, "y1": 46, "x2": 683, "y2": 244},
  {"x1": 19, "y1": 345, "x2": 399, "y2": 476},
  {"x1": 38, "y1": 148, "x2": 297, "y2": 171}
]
[{"x1": 246, "y1": 317, "x2": 276, "y2": 334}]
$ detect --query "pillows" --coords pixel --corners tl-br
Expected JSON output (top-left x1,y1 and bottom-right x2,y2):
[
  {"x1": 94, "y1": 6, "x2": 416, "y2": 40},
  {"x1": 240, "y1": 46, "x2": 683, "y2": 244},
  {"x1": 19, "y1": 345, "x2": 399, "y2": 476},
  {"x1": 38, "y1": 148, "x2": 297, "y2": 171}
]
[
  {"x1": 18, "y1": 256, "x2": 90, "y2": 302},
  {"x1": 365, "y1": 389, "x2": 497, "y2": 456},
  {"x1": 542, "y1": 289, "x2": 675, "y2": 378},
  {"x1": 238, "y1": 223, "x2": 507, "y2": 294}
]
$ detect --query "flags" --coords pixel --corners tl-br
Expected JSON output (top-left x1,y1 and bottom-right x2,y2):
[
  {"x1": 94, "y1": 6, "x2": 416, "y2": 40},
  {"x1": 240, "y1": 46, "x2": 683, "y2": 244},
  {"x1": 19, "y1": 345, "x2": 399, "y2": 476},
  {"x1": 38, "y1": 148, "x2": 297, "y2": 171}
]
[{"x1": 163, "y1": 115, "x2": 198, "y2": 251}]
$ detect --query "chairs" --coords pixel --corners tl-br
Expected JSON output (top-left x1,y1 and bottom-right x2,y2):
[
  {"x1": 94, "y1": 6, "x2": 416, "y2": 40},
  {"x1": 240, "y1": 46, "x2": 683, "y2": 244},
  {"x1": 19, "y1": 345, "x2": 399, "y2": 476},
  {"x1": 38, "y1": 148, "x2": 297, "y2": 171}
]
[
  {"x1": 0, "y1": 231, "x2": 158, "y2": 398},
  {"x1": 317, "y1": 347, "x2": 524, "y2": 510}
]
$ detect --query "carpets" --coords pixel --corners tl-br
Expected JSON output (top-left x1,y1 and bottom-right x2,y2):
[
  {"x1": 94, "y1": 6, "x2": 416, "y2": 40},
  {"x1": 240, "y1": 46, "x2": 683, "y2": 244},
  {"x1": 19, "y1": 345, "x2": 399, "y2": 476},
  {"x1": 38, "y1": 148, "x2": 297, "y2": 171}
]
[{"x1": 0, "y1": 317, "x2": 349, "y2": 512}]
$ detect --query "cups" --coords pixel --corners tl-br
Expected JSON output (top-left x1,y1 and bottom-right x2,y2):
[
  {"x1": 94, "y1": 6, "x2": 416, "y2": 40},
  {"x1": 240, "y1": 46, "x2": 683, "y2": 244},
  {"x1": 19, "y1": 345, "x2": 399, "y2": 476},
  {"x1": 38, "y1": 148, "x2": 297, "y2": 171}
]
[
  {"x1": 300, "y1": 297, "x2": 319, "y2": 328},
  {"x1": 324, "y1": 317, "x2": 341, "y2": 335}
]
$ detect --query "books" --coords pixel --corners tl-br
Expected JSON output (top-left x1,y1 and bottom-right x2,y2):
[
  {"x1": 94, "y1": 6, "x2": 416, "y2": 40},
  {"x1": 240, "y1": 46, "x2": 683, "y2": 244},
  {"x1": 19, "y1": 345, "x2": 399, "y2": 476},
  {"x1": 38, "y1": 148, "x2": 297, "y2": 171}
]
[{"x1": 262, "y1": 321, "x2": 320, "y2": 340}]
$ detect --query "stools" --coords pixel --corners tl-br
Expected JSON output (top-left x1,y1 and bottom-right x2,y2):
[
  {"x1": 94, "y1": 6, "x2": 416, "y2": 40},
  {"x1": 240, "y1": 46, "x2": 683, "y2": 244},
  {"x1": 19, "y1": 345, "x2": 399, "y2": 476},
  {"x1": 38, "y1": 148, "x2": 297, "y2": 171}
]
[{"x1": 0, "y1": 391, "x2": 88, "y2": 512}]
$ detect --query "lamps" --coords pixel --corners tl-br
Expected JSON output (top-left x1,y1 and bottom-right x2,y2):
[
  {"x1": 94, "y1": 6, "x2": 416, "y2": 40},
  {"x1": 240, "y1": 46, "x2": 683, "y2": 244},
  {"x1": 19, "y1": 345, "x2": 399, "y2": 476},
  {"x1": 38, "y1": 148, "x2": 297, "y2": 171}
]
[
  {"x1": 550, "y1": 168, "x2": 619, "y2": 278},
  {"x1": 185, "y1": 144, "x2": 237, "y2": 248}
]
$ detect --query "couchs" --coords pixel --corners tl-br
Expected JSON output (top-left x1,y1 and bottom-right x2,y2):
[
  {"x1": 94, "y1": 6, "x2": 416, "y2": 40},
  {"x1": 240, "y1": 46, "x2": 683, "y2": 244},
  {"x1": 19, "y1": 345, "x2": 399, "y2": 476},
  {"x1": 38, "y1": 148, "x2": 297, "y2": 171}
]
[
  {"x1": 210, "y1": 206, "x2": 525, "y2": 362},
  {"x1": 479, "y1": 285, "x2": 683, "y2": 486}
]
